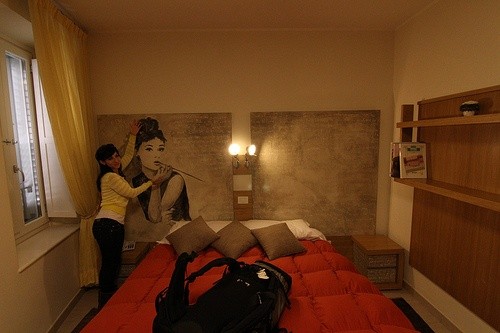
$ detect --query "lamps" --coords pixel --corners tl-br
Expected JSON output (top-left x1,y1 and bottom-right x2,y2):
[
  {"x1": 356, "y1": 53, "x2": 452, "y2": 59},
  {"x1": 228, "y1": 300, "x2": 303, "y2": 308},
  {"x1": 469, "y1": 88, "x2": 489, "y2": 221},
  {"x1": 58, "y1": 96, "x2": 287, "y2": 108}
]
[{"x1": 229, "y1": 144, "x2": 256, "y2": 169}]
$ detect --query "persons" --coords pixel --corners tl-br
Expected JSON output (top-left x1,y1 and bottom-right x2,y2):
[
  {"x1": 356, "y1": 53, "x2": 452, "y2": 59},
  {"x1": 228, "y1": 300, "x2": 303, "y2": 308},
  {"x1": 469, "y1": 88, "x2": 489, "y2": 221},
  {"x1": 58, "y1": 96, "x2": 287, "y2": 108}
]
[{"x1": 92, "y1": 119, "x2": 166, "y2": 311}]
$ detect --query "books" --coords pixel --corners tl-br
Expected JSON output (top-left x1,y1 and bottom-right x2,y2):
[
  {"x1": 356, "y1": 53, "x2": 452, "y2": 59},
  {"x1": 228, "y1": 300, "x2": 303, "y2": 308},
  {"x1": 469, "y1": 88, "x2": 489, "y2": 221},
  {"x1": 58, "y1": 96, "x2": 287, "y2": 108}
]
[{"x1": 389, "y1": 142, "x2": 427, "y2": 179}]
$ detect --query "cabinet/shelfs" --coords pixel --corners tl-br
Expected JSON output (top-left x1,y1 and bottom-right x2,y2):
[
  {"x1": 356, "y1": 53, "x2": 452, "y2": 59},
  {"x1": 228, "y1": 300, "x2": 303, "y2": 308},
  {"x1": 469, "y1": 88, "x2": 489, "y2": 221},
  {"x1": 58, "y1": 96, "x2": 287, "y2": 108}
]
[{"x1": 392, "y1": 113, "x2": 500, "y2": 214}]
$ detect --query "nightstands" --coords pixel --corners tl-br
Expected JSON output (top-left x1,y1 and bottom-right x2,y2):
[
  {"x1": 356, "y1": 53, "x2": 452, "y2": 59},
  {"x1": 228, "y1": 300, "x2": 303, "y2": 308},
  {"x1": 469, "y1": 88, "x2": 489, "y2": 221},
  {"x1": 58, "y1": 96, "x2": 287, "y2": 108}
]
[
  {"x1": 351, "y1": 234, "x2": 405, "y2": 291},
  {"x1": 115, "y1": 242, "x2": 148, "y2": 289}
]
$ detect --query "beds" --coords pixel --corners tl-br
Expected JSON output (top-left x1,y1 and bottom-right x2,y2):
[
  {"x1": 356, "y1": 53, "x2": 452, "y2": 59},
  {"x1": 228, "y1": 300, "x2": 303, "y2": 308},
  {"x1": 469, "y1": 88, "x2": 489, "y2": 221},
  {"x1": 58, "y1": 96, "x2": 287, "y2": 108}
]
[{"x1": 71, "y1": 219, "x2": 419, "y2": 333}]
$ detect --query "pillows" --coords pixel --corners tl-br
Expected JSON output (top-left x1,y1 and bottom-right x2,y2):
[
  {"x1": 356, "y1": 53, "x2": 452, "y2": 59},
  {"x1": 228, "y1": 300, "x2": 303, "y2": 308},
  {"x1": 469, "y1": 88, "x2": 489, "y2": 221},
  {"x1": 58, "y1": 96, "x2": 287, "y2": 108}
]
[
  {"x1": 156, "y1": 219, "x2": 233, "y2": 245},
  {"x1": 208, "y1": 217, "x2": 259, "y2": 260},
  {"x1": 243, "y1": 219, "x2": 327, "y2": 240},
  {"x1": 250, "y1": 223, "x2": 308, "y2": 261},
  {"x1": 166, "y1": 216, "x2": 221, "y2": 260}
]
